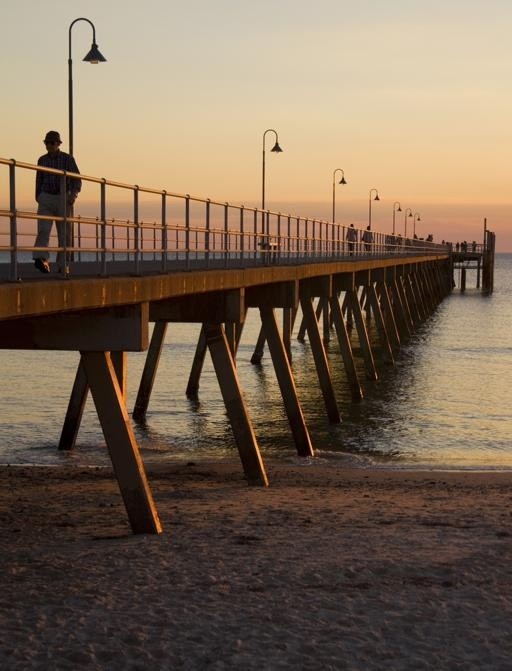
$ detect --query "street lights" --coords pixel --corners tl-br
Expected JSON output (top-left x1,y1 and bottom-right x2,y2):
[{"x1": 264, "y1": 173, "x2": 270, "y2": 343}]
[
  {"x1": 65, "y1": 17, "x2": 107, "y2": 265},
  {"x1": 404, "y1": 208, "x2": 413, "y2": 238},
  {"x1": 392, "y1": 202, "x2": 402, "y2": 236},
  {"x1": 367, "y1": 189, "x2": 381, "y2": 230},
  {"x1": 260, "y1": 129, "x2": 284, "y2": 263},
  {"x1": 331, "y1": 168, "x2": 348, "y2": 257},
  {"x1": 413, "y1": 213, "x2": 421, "y2": 238}
]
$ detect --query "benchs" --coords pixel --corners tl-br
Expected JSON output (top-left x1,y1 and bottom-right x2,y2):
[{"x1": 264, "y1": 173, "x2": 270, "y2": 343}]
[{"x1": 258, "y1": 242, "x2": 284, "y2": 261}]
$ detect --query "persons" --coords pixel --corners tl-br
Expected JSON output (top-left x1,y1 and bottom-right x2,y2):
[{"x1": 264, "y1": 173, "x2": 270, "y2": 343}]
[
  {"x1": 31, "y1": 130, "x2": 81, "y2": 276},
  {"x1": 362, "y1": 225, "x2": 374, "y2": 256},
  {"x1": 346, "y1": 223, "x2": 357, "y2": 257},
  {"x1": 385, "y1": 234, "x2": 433, "y2": 255},
  {"x1": 441, "y1": 240, "x2": 477, "y2": 253}
]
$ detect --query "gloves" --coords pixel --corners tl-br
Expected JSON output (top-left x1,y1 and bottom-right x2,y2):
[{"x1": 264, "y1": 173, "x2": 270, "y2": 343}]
[{"x1": 69, "y1": 191, "x2": 77, "y2": 204}]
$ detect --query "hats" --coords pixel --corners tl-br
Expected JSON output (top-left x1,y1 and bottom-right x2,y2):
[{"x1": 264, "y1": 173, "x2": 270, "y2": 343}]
[{"x1": 43, "y1": 131, "x2": 62, "y2": 143}]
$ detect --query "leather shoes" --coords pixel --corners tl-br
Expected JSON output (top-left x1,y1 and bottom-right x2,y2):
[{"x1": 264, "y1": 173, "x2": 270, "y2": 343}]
[
  {"x1": 58, "y1": 266, "x2": 69, "y2": 273},
  {"x1": 35, "y1": 258, "x2": 50, "y2": 273}
]
